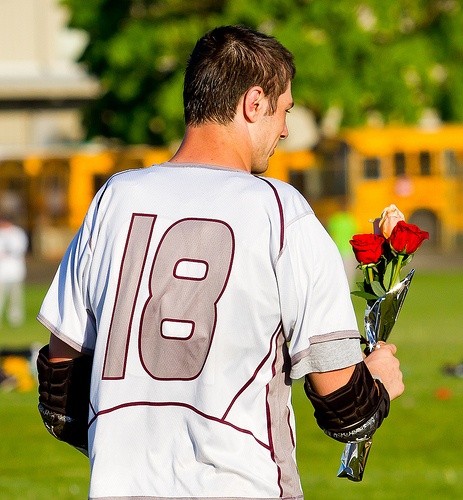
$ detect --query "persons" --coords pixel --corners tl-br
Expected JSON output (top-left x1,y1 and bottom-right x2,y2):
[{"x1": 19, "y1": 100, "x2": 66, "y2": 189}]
[
  {"x1": 0, "y1": 213, "x2": 28, "y2": 326},
  {"x1": 36, "y1": 25, "x2": 405, "y2": 500}
]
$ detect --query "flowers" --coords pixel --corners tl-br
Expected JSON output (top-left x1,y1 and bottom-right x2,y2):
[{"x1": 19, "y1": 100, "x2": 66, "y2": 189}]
[{"x1": 335, "y1": 204, "x2": 430, "y2": 482}]
